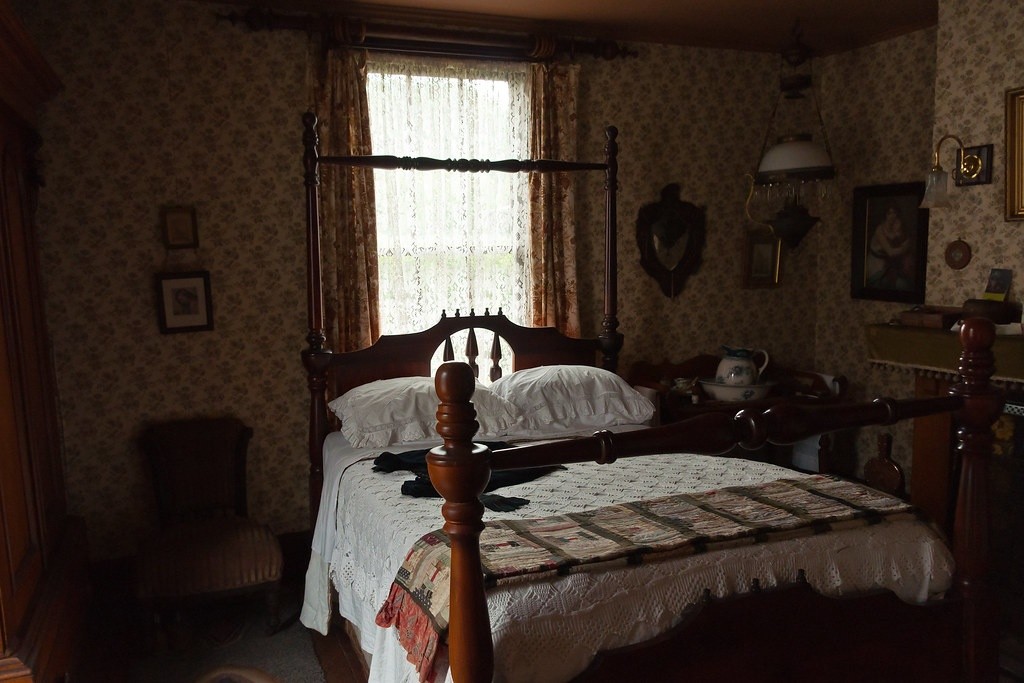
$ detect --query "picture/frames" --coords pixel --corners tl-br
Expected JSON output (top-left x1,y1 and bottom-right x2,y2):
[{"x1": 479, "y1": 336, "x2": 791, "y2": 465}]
[
  {"x1": 851, "y1": 180, "x2": 929, "y2": 307},
  {"x1": 742, "y1": 227, "x2": 784, "y2": 292},
  {"x1": 1005, "y1": 87, "x2": 1024, "y2": 222},
  {"x1": 156, "y1": 270, "x2": 214, "y2": 334},
  {"x1": 160, "y1": 204, "x2": 200, "y2": 250}
]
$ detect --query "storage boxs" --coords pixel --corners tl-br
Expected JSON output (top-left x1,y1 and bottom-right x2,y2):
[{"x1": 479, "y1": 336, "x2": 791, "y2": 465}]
[{"x1": 900, "y1": 306, "x2": 964, "y2": 331}]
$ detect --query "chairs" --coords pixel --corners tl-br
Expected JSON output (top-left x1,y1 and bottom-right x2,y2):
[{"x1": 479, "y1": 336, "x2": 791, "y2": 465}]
[{"x1": 126, "y1": 414, "x2": 285, "y2": 657}]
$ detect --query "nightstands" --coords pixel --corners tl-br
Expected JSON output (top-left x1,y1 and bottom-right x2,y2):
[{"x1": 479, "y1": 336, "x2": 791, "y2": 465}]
[{"x1": 625, "y1": 354, "x2": 835, "y2": 479}]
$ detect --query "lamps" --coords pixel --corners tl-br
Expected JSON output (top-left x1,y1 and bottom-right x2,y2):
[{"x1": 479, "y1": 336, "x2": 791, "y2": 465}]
[
  {"x1": 743, "y1": 15, "x2": 836, "y2": 251},
  {"x1": 919, "y1": 134, "x2": 994, "y2": 209}
]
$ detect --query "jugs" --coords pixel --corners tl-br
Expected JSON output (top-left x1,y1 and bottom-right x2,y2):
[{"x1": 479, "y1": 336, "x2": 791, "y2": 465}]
[{"x1": 715, "y1": 344, "x2": 769, "y2": 385}]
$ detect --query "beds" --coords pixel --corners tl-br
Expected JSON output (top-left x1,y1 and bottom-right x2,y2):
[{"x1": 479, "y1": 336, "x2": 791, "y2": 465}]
[{"x1": 298, "y1": 107, "x2": 998, "y2": 683}]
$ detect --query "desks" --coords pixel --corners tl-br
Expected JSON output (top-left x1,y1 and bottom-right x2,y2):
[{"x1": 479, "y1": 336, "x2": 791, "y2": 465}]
[{"x1": 866, "y1": 322, "x2": 1024, "y2": 539}]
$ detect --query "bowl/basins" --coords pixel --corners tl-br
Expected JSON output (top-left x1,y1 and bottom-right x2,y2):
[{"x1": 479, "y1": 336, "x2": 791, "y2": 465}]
[{"x1": 697, "y1": 377, "x2": 779, "y2": 401}]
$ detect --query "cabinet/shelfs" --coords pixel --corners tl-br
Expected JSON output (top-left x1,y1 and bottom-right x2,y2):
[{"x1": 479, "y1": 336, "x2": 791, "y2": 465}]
[{"x1": 0, "y1": 0, "x2": 98, "y2": 683}]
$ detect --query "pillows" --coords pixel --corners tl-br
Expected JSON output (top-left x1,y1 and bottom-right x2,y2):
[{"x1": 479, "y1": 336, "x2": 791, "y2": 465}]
[{"x1": 327, "y1": 365, "x2": 659, "y2": 451}]
